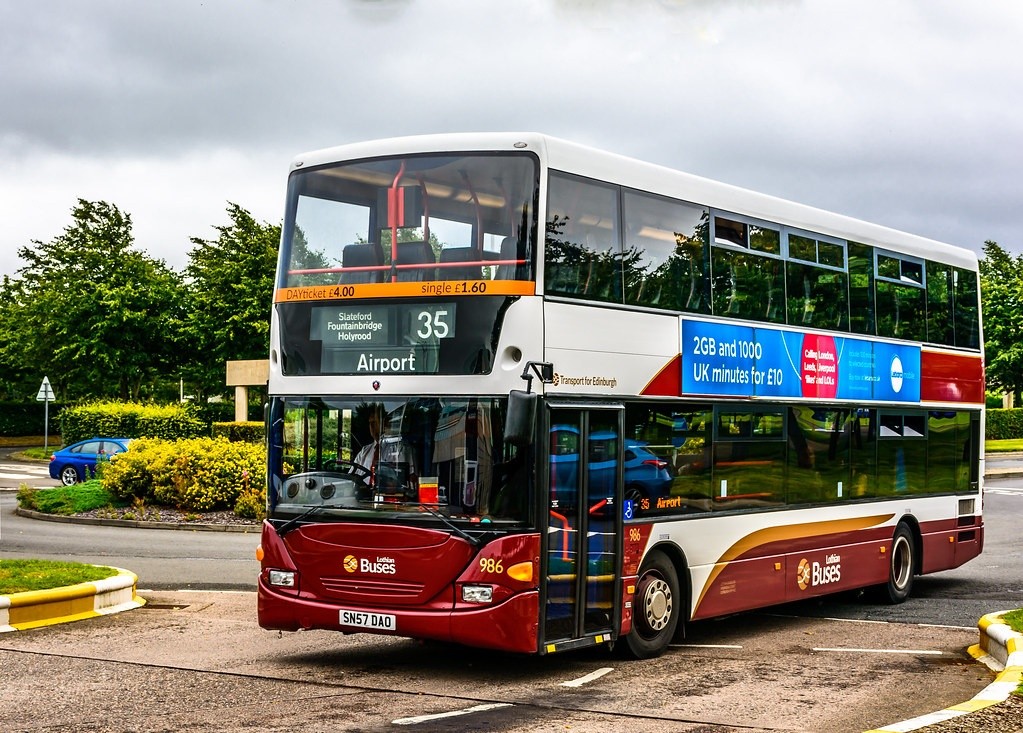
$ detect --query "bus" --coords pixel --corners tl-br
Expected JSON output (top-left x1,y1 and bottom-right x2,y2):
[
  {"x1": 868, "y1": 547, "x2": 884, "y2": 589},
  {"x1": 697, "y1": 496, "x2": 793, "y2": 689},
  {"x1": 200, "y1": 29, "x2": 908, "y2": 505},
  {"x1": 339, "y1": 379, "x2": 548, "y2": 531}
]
[{"x1": 254, "y1": 132, "x2": 989, "y2": 659}]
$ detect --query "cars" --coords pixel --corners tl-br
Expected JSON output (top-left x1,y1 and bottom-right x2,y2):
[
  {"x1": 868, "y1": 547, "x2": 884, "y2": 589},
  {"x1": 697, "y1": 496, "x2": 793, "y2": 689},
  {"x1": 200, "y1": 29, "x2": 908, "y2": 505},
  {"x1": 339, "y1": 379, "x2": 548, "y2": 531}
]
[{"x1": 48, "y1": 439, "x2": 133, "y2": 486}]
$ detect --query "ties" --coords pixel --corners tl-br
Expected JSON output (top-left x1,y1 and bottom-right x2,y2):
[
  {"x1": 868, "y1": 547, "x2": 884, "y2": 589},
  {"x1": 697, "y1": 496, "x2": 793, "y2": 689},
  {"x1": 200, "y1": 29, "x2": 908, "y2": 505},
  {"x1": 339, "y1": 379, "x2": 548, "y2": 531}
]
[{"x1": 371, "y1": 445, "x2": 381, "y2": 486}]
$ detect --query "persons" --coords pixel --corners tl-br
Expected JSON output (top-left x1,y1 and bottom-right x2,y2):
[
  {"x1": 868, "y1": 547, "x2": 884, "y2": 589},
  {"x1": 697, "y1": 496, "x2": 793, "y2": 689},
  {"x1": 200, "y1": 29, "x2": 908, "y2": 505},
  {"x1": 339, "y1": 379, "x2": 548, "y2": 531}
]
[{"x1": 346, "y1": 411, "x2": 419, "y2": 498}]
